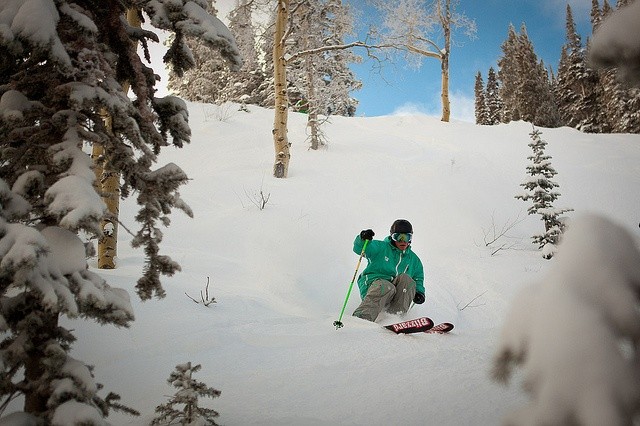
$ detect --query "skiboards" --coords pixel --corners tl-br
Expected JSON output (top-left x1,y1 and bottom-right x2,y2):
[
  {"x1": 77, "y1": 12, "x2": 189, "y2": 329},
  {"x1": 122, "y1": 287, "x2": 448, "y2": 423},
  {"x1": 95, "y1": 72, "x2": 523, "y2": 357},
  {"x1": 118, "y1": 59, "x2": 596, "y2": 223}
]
[{"x1": 379, "y1": 317, "x2": 454, "y2": 335}]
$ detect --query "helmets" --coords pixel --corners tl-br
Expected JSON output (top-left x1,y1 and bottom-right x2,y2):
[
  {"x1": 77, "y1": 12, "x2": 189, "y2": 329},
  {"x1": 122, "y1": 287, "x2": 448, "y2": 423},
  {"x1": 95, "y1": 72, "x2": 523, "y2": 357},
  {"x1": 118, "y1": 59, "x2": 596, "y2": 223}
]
[{"x1": 391, "y1": 220, "x2": 413, "y2": 243}]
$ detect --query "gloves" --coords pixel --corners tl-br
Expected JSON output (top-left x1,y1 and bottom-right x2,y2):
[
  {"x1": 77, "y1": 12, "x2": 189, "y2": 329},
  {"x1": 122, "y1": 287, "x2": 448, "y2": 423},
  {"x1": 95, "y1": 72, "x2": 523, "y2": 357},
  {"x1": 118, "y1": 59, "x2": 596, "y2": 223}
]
[
  {"x1": 361, "y1": 229, "x2": 374, "y2": 240},
  {"x1": 413, "y1": 292, "x2": 425, "y2": 304}
]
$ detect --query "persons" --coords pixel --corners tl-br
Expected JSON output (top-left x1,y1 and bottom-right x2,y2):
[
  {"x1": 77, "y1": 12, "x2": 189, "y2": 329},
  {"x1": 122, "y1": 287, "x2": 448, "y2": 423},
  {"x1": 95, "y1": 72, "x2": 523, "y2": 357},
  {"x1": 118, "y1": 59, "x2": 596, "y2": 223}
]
[{"x1": 351, "y1": 218, "x2": 426, "y2": 322}]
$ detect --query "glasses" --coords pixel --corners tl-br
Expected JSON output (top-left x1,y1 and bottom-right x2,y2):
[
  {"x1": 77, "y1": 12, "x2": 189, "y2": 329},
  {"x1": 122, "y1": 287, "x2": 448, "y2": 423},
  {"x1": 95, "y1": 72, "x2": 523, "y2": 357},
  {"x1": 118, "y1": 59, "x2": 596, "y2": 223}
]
[{"x1": 390, "y1": 232, "x2": 413, "y2": 242}]
